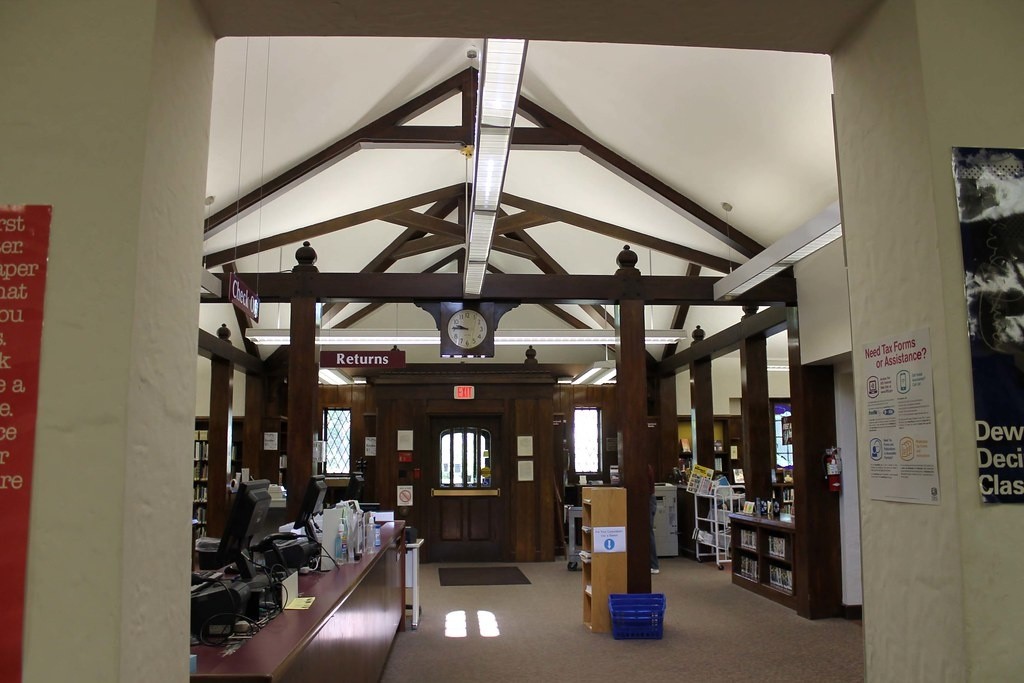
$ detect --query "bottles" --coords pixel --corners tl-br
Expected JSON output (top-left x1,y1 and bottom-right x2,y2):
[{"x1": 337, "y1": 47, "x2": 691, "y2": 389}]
[{"x1": 335, "y1": 525, "x2": 347, "y2": 565}]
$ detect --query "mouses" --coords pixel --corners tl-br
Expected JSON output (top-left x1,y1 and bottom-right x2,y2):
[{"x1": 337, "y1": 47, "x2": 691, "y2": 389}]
[{"x1": 224, "y1": 565, "x2": 240, "y2": 575}]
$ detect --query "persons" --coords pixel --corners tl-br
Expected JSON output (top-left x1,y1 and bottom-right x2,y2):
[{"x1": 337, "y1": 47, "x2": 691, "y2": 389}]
[{"x1": 647, "y1": 465, "x2": 660, "y2": 573}]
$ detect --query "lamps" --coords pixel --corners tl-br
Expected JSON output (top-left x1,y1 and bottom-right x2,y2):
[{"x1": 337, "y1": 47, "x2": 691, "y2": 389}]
[
  {"x1": 713, "y1": 198, "x2": 842, "y2": 302},
  {"x1": 458, "y1": 37, "x2": 529, "y2": 296},
  {"x1": 245, "y1": 247, "x2": 688, "y2": 345}
]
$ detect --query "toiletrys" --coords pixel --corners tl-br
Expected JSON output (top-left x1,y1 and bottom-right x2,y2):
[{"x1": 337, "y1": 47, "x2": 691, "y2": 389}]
[
  {"x1": 366, "y1": 511, "x2": 382, "y2": 555},
  {"x1": 335, "y1": 516, "x2": 348, "y2": 566}
]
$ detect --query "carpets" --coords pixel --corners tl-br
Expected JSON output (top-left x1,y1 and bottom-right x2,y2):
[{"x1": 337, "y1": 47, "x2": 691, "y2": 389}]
[{"x1": 438, "y1": 566, "x2": 532, "y2": 586}]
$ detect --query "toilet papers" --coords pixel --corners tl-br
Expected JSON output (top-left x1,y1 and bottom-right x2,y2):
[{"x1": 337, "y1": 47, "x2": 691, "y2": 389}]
[{"x1": 320, "y1": 509, "x2": 343, "y2": 571}]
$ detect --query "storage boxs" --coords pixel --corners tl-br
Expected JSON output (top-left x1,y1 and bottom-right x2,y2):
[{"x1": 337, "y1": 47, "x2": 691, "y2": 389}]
[
  {"x1": 609, "y1": 592, "x2": 667, "y2": 640},
  {"x1": 200, "y1": 430, "x2": 209, "y2": 440},
  {"x1": 195, "y1": 430, "x2": 200, "y2": 440}
]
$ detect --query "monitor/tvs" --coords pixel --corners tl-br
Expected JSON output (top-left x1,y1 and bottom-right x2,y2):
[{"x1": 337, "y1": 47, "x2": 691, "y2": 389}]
[
  {"x1": 215, "y1": 479, "x2": 271, "y2": 570},
  {"x1": 344, "y1": 471, "x2": 365, "y2": 503},
  {"x1": 294, "y1": 474, "x2": 327, "y2": 529}
]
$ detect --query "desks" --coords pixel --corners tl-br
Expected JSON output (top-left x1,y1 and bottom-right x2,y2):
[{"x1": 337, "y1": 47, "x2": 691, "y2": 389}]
[{"x1": 406, "y1": 538, "x2": 425, "y2": 631}]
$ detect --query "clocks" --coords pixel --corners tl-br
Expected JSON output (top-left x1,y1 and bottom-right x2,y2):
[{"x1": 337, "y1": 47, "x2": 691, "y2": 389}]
[{"x1": 448, "y1": 309, "x2": 488, "y2": 348}]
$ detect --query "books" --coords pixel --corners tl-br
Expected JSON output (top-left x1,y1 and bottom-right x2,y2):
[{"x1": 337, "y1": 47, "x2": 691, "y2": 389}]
[
  {"x1": 770, "y1": 565, "x2": 792, "y2": 591},
  {"x1": 768, "y1": 535, "x2": 790, "y2": 559},
  {"x1": 740, "y1": 556, "x2": 758, "y2": 578},
  {"x1": 740, "y1": 529, "x2": 757, "y2": 549},
  {"x1": 192, "y1": 442, "x2": 208, "y2": 537}
]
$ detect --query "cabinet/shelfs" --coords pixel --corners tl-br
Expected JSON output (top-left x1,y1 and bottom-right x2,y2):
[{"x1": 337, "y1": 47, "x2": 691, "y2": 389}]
[
  {"x1": 245, "y1": 378, "x2": 288, "y2": 486},
  {"x1": 581, "y1": 487, "x2": 628, "y2": 634},
  {"x1": 727, "y1": 512, "x2": 797, "y2": 611},
  {"x1": 193, "y1": 416, "x2": 246, "y2": 564},
  {"x1": 693, "y1": 484, "x2": 746, "y2": 570},
  {"x1": 677, "y1": 417, "x2": 733, "y2": 484}
]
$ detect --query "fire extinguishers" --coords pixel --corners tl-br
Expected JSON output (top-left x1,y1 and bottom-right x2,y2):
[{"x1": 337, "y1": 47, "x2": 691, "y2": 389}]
[{"x1": 821, "y1": 447, "x2": 842, "y2": 492}]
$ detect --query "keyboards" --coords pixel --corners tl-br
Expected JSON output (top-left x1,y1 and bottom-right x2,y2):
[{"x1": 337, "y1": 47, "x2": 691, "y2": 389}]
[
  {"x1": 191, "y1": 571, "x2": 223, "y2": 594},
  {"x1": 273, "y1": 538, "x2": 297, "y2": 547}
]
